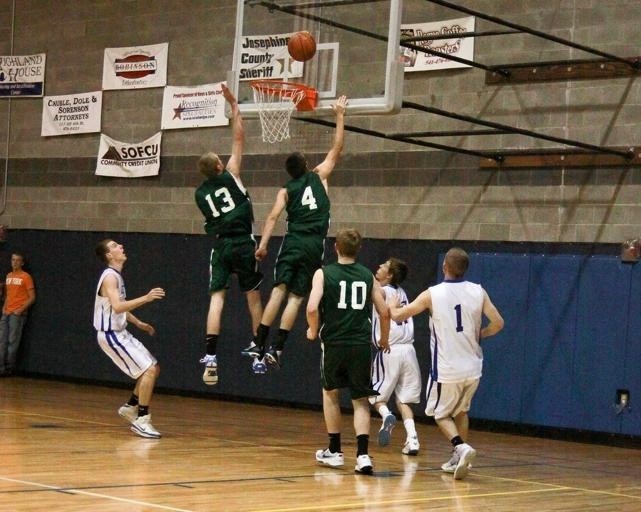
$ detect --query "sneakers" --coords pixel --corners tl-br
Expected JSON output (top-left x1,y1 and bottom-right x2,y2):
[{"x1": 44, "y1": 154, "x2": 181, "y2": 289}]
[
  {"x1": 402, "y1": 435, "x2": 420, "y2": 454},
  {"x1": 240, "y1": 336, "x2": 263, "y2": 357},
  {"x1": 315, "y1": 447, "x2": 344, "y2": 469},
  {"x1": 118, "y1": 403, "x2": 139, "y2": 423},
  {"x1": 440, "y1": 455, "x2": 472, "y2": 473},
  {"x1": 265, "y1": 349, "x2": 282, "y2": 369},
  {"x1": 354, "y1": 454, "x2": 373, "y2": 473},
  {"x1": 202, "y1": 357, "x2": 218, "y2": 385},
  {"x1": 128, "y1": 413, "x2": 161, "y2": 439},
  {"x1": 453, "y1": 443, "x2": 476, "y2": 479},
  {"x1": 249, "y1": 356, "x2": 268, "y2": 375},
  {"x1": 377, "y1": 412, "x2": 396, "y2": 448}
]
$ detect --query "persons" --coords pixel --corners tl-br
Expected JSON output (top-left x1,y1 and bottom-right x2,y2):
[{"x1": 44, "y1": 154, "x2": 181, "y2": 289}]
[
  {"x1": 390, "y1": 248, "x2": 505, "y2": 480},
  {"x1": 305, "y1": 230, "x2": 390, "y2": 474},
  {"x1": 195, "y1": 85, "x2": 267, "y2": 384},
  {"x1": 241, "y1": 96, "x2": 349, "y2": 370},
  {"x1": 0, "y1": 252, "x2": 35, "y2": 377},
  {"x1": 94, "y1": 239, "x2": 165, "y2": 439},
  {"x1": 368, "y1": 257, "x2": 421, "y2": 455}
]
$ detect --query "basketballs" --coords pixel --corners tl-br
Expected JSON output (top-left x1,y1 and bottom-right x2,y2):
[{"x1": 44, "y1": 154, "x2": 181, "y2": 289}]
[{"x1": 288, "y1": 32, "x2": 316, "y2": 62}]
